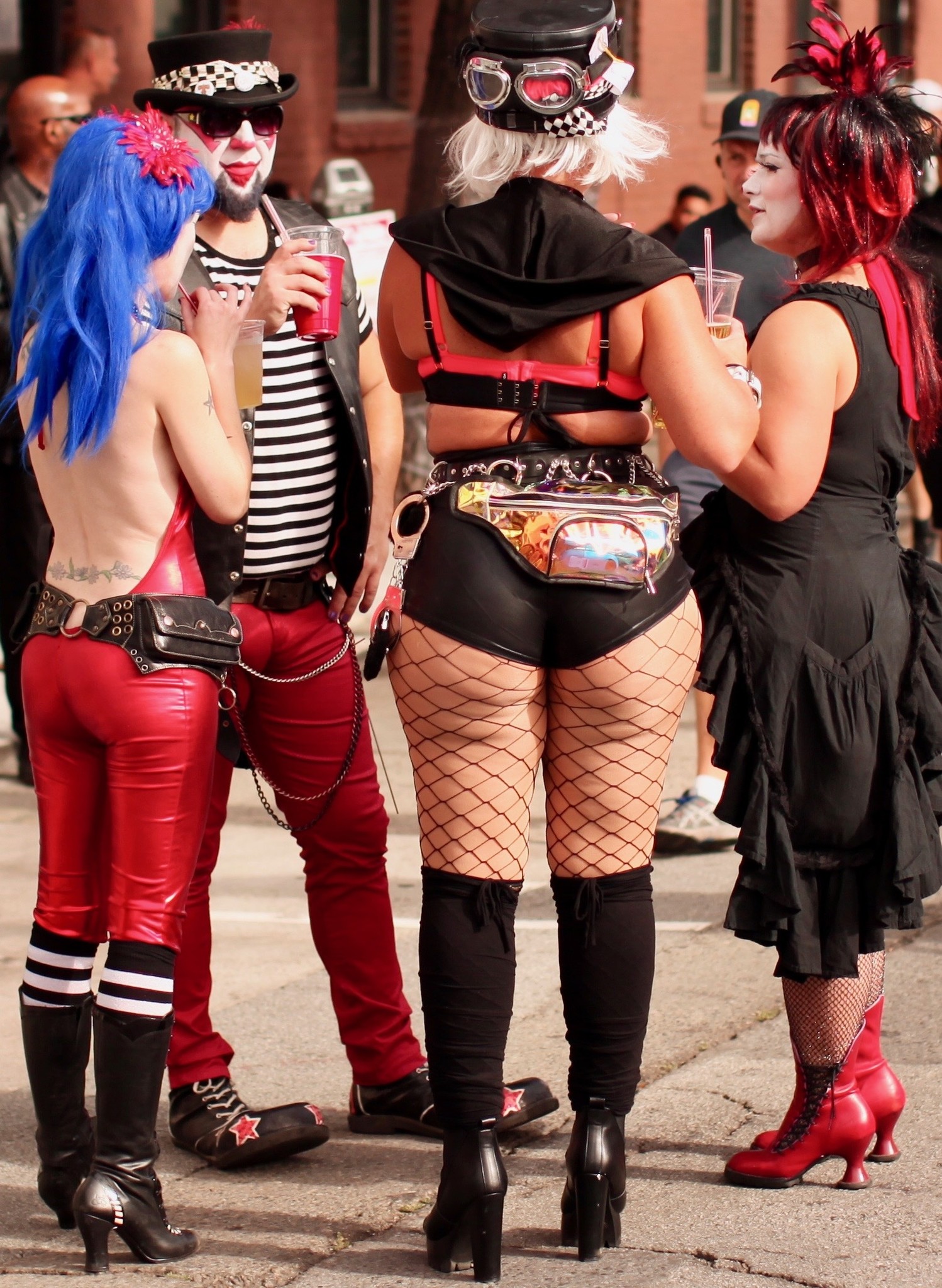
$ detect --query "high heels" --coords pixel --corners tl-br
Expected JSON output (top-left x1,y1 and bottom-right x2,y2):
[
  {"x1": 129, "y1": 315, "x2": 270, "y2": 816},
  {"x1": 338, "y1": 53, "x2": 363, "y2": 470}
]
[
  {"x1": 559, "y1": 1097, "x2": 628, "y2": 1262},
  {"x1": 422, "y1": 1118, "x2": 508, "y2": 1280}
]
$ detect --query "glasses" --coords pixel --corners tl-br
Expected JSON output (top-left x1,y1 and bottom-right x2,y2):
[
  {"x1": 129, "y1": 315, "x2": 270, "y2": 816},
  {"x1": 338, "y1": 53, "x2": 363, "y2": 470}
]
[
  {"x1": 464, "y1": 53, "x2": 588, "y2": 117},
  {"x1": 175, "y1": 104, "x2": 283, "y2": 137},
  {"x1": 39, "y1": 115, "x2": 92, "y2": 126}
]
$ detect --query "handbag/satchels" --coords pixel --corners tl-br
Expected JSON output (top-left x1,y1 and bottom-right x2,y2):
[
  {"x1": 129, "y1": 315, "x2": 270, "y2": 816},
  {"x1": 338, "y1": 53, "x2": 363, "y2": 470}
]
[{"x1": 450, "y1": 474, "x2": 679, "y2": 588}]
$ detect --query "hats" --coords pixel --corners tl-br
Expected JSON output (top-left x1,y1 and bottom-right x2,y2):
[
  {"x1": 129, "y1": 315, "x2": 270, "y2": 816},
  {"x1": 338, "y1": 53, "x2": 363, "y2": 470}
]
[
  {"x1": 465, "y1": 0, "x2": 620, "y2": 131},
  {"x1": 710, "y1": 89, "x2": 785, "y2": 145},
  {"x1": 135, "y1": 28, "x2": 299, "y2": 115}
]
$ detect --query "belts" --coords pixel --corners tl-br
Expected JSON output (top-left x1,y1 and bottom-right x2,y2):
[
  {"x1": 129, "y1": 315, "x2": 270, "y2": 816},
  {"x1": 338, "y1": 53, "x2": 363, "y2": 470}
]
[{"x1": 436, "y1": 450, "x2": 647, "y2": 484}]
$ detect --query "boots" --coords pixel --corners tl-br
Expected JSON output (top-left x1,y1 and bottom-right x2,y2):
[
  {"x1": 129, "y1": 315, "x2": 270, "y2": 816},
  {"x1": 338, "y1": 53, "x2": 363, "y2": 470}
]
[
  {"x1": 18, "y1": 983, "x2": 96, "y2": 1230},
  {"x1": 73, "y1": 1000, "x2": 197, "y2": 1272},
  {"x1": 723, "y1": 1018, "x2": 876, "y2": 1190},
  {"x1": 750, "y1": 994, "x2": 908, "y2": 1160}
]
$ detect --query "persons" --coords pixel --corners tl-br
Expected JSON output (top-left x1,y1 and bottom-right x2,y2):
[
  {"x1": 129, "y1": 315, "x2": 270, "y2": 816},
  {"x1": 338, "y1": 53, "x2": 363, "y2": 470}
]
[
  {"x1": 0, "y1": 116, "x2": 255, "y2": 1279},
  {"x1": 642, "y1": 78, "x2": 942, "y2": 932},
  {"x1": 678, "y1": 0, "x2": 942, "y2": 1191},
  {"x1": 0, "y1": 27, "x2": 117, "y2": 786},
  {"x1": 375, "y1": 0, "x2": 763, "y2": 1283},
  {"x1": 134, "y1": 36, "x2": 558, "y2": 1161}
]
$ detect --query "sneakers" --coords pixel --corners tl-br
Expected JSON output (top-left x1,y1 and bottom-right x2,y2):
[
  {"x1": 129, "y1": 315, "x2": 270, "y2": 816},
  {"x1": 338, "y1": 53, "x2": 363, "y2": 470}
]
[
  {"x1": 649, "y1": 789, "x2": 743, "y2": 856},
  {"x1": 347, "y1": 1061, "x2": 562, "y2": 1139},
  {"x1": 169, "y1": 1080, "x2": 330, "y2": 1168}
]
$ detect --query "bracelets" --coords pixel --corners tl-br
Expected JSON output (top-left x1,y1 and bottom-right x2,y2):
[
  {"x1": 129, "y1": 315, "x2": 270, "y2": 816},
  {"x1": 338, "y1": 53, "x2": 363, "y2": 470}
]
[{"x1": 724, "y1": 362, "x2": 762, "y2": 409}]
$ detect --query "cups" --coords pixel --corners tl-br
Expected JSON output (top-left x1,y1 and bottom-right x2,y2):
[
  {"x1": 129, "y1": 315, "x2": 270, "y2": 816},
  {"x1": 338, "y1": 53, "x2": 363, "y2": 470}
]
[
  {"x1": 689, "y1": 266, "x2": 744, "y2": 339},
  {"x1": 233, "y1": 319, "x2": 266, "y2": 409},
  {"x1": 280, "y1": 225, "x2": 345, "y2": 342}
]
